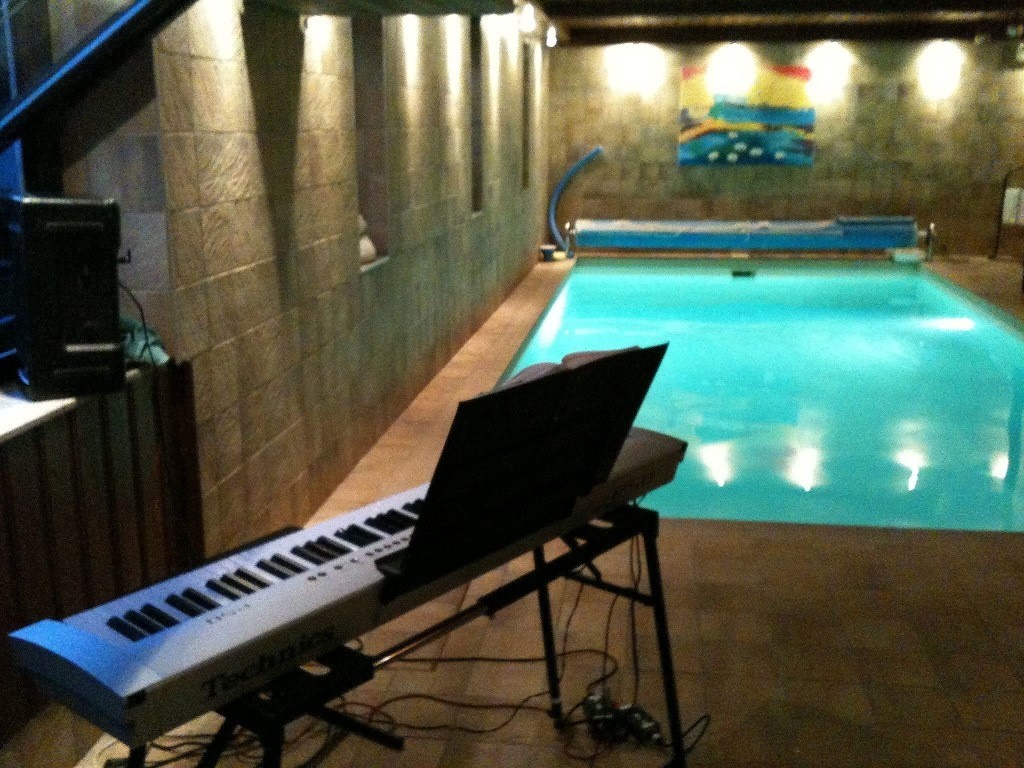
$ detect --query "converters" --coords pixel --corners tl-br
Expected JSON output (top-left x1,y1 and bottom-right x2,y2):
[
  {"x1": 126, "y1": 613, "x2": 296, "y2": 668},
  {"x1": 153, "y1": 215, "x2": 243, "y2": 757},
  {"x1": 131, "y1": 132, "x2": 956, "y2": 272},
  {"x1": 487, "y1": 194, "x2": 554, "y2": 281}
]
[{"x1": 580, "y1": 695, "x2": 663, "y2": 743}]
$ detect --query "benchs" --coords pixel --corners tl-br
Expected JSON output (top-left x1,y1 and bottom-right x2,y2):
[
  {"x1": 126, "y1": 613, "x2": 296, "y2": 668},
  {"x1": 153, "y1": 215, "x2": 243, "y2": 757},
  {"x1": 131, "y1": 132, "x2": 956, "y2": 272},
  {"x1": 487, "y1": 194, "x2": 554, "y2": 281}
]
[{"x1": 574, "y1": 217, "x2": 920, "y2": 254}]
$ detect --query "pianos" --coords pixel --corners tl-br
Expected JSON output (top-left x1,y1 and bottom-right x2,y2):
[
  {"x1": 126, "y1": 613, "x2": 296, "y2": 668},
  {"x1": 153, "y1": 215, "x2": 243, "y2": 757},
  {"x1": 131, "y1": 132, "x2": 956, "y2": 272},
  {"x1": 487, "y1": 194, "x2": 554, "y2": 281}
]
[{"x1": 8, "y1": 423, "x2": 691, "y2": 768}]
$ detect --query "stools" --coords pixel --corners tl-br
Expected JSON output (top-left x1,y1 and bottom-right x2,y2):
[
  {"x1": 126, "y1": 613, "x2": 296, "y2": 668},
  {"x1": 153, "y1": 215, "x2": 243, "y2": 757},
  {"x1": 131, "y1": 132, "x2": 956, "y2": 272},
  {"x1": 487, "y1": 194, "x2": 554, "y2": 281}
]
[{"x1": 168, "y1": 526, "x2": 405, "y2": 768}]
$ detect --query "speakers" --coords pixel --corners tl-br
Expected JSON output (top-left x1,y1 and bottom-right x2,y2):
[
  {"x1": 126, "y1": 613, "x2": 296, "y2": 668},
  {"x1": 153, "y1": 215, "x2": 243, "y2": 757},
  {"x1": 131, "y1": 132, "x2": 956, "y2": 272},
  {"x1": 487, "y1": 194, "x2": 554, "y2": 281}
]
[{"x1": 0, "y1": 195, "x2": 124, "y2": 402}]
